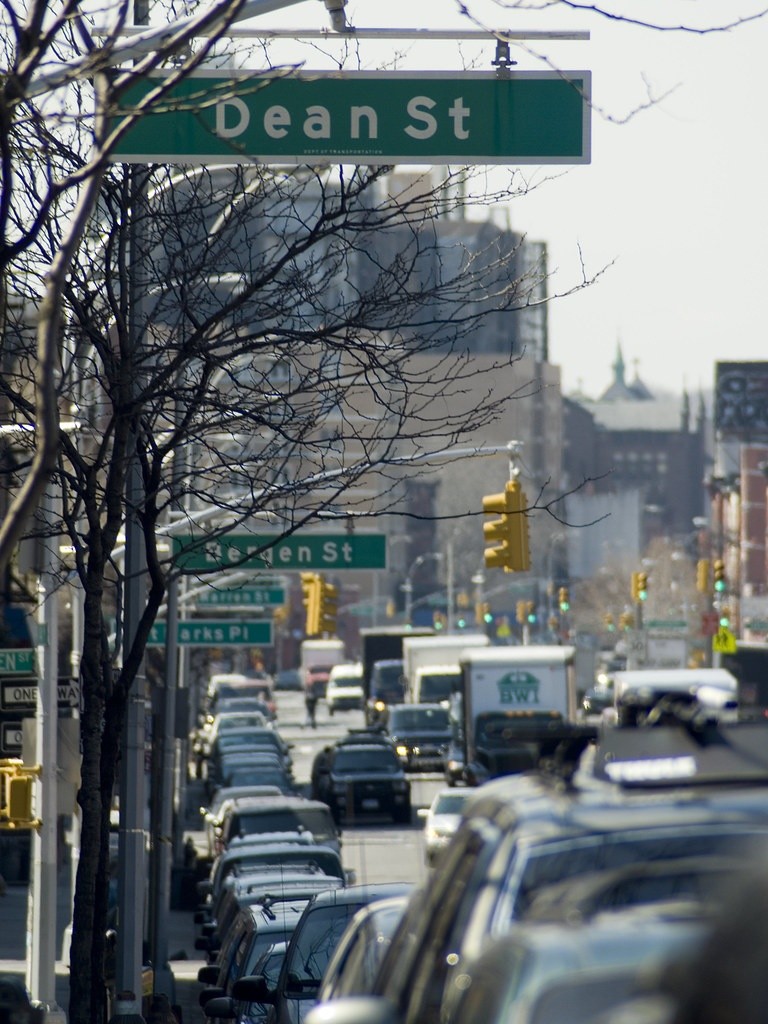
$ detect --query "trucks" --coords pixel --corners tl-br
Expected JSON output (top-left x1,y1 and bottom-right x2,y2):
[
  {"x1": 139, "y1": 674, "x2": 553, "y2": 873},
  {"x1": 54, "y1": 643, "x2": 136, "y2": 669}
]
[
  {"x1": 327, "y1": 626, "x2": 600, "y2": 787},
  {"x1": 300, "y1": 641, "x2": 343, "y2": 685}
]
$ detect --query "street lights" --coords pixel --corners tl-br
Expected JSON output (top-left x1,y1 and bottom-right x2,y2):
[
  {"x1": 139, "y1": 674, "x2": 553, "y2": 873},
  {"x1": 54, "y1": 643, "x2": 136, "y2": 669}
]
[
  {"x1": 69, "y1": 271, "x2": 255, "y2": 676},
  {"x1": 405, "y1": 552, "x2": 443, "y2": 624}
]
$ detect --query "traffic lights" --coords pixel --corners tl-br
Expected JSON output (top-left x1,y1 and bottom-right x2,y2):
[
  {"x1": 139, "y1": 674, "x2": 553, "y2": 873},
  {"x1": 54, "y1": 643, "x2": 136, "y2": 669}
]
[
  {"x1": 475, "y1": 588, "x2": 570, "y2": 624},
  {"x1": 605, "y1": 614, "x2": 633, "y2": 632},
  {"x1": 7, "y1": 776, "x2": 38, "y2": 821},
  {"x1": 631, "y1": 560, "x2": 725, "y2": 601},
  {"x1": 480, "y1": 481, "x2": 531, "y2": 574},
  {"x1": 300, "y1": 573, "x2": 338, "y2": 635}
]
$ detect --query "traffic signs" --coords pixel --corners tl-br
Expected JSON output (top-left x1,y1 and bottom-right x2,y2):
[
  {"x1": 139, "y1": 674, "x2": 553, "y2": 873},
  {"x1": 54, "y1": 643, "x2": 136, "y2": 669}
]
[
  {"x1": 0, "y1": 648, "x2": 35, "y2": 675},
  {"x1": 0, "y1": 678, "x2": 80, "y2": 753},
  {"x1": 94, "y1": 69, "x2": 591, "y2": 167},
  {"x1": 171, "y1": 532, "x2": 386, "y2": 570},
  {"x1": 196, "y1": 585, "x2": 285, "y2": 607}
]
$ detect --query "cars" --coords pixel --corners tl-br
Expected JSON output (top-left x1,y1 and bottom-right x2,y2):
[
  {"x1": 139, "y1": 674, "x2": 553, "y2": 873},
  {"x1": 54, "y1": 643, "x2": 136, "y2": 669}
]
[
  {"x1": 199, "y1": 674, "x2": 417, "y2": 1024},
  {"x1": 415, "y1": 668, "x2": 767, "y2": 868},
  {"x1": 298, "y1": 773, "x2": 768, "y2": 1024},
  {"x1": 304, "y1": 664, "x2": 332, "y2": 699}
]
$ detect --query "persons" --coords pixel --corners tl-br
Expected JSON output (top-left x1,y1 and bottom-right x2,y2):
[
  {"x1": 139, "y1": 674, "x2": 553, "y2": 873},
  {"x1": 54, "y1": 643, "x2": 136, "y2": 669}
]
[{"x1": 305, "y1": 689, "x2": 318, "y2": 727}]
